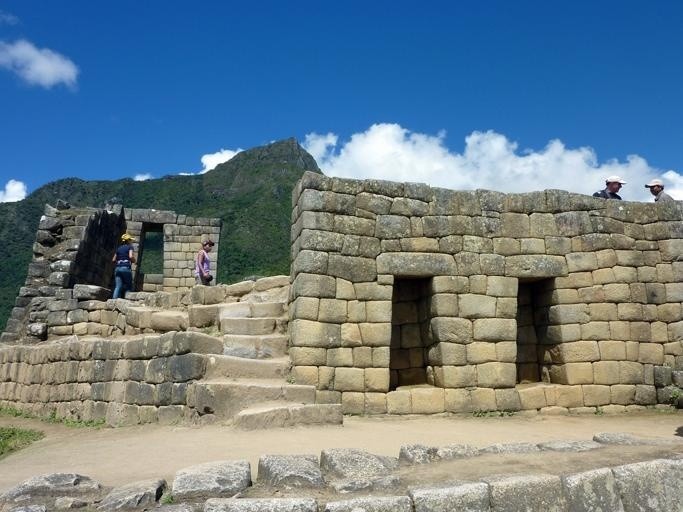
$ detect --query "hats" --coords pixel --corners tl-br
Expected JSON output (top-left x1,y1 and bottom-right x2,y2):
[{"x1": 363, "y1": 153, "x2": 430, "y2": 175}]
[
  {"x1": 606, "y1": 176, "x2": 626, "y2": 184},
  {"x1": 203, "y1": 240, "x2": 214, "y2": 246},
  {"x1": 645, "y1": 179, "x2": 664, "y2": 187},
  {"x1": 121, "y1": 234, "x2": 135, "y2": 242}
]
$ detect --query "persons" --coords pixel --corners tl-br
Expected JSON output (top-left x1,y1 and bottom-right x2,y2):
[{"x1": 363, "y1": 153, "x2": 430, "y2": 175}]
[
  {"x1": 644, "y1": 179, "x2": 673, "y2": 201},
  {"x1": 592, "y1": 176, "x2": 627, "y2": 200},
  {"x1": 110, "y1": 233, "x2": 136, "y2": 299},
  {"x1": 194, "y1": 240, "x2": 214, "y2": 286}
]
